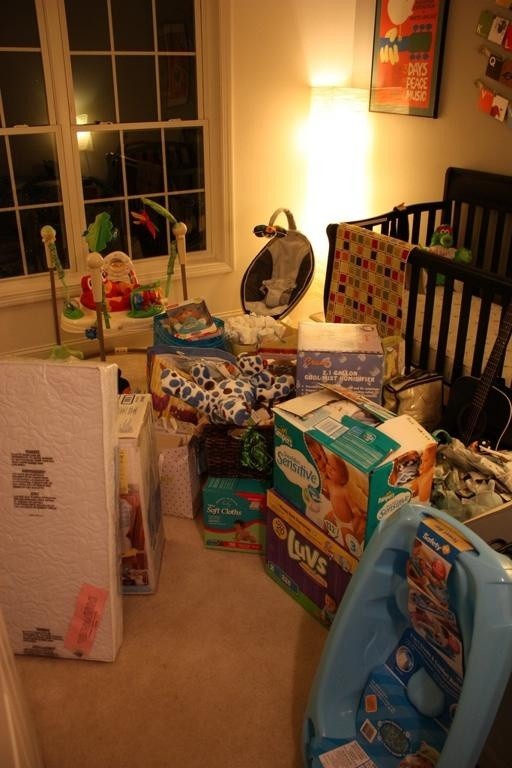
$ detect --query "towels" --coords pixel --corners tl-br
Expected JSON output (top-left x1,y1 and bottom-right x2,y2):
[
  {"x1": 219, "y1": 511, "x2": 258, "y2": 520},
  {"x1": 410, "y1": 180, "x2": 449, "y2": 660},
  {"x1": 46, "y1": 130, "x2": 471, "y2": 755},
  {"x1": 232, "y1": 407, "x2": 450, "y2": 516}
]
[{"x1": 328, "y1": 214, "x2": 425, "y2": 341}]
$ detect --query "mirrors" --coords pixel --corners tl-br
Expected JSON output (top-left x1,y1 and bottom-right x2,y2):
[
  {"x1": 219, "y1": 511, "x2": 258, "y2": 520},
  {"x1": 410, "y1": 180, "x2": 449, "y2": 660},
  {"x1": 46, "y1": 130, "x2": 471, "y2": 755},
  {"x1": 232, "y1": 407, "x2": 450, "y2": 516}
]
[{"x1": 238, "y1": 226, "x2": 314, "y2": 325}]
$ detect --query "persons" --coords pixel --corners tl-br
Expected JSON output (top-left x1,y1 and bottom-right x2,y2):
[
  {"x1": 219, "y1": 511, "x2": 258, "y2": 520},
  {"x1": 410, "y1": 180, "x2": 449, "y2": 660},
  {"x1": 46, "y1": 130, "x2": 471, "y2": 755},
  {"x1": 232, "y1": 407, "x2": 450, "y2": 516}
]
[
  {"x1": 234, "y1": 518, "x2": 257, "y2": 543},
  {"x1": 304, "y1": 435, "x2": 329, "y2": 485},
  {"x1": 410, "y1": 441, "x2": 437, "y2": 503},
  {"x1": 318, "y1": 589, "x2": 340, "y2": 624},
  {"x1": 426, "y1": 556, "x2": 451, "y2": 590},
  {"x1": 323, "y1": 455, "x2": 365, "y2": 550}
]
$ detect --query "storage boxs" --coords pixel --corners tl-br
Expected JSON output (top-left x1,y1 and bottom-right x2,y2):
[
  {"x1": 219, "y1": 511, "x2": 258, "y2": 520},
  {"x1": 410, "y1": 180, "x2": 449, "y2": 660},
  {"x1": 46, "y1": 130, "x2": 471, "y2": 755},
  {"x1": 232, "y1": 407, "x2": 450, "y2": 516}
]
[{"x1": 111, "y1": 389, "x2": 172, "y2": 593}]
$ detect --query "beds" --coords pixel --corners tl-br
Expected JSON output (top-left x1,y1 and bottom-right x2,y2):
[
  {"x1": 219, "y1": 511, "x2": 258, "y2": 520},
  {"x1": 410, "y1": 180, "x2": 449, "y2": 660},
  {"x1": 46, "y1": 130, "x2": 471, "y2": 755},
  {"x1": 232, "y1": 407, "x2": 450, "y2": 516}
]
[{"x1": 321, "y1": 168, "x2": 510, "y2": 399}]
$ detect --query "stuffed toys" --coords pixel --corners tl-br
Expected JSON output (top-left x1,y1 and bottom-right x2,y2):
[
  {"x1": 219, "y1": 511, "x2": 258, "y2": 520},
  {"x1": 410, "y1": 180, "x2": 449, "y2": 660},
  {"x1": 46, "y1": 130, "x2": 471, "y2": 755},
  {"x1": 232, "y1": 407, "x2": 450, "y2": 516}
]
[
  {"x1": 417, "y1": 222, "x2": 474, "y2": 285},
  {"x1": 160, "y1": 348, "x2": 297, "y2": 426}
]
